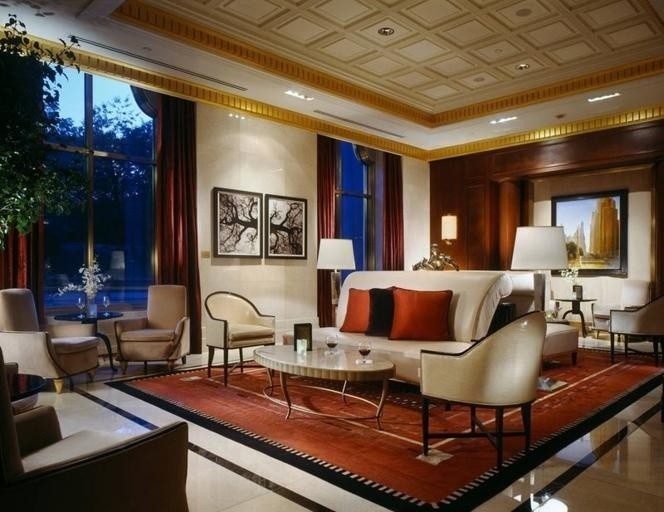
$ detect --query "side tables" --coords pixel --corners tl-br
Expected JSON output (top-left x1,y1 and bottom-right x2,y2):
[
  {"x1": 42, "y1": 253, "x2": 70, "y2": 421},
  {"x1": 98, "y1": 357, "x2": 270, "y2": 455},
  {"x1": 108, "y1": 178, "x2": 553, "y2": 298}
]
[{"x1": 553, "y1": 296, "x2": 597, "y2": 337}]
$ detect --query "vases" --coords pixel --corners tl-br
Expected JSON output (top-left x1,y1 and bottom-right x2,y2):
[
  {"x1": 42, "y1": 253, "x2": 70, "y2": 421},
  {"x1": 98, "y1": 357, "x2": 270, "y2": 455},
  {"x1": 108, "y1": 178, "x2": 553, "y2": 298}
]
[{"x1": 571, "y1": 285, "x2": 583, "y2": 299}]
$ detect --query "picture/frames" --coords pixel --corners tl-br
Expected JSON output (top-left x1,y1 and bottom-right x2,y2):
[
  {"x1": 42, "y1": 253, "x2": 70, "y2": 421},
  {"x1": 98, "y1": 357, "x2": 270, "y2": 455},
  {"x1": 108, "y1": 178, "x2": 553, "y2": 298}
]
[
  {"x1": 212, "y1": 187, "x2": 307, "y2": 261},
  {"x1": 549, "y1": 187, "x2": 629, "y2": 278}
]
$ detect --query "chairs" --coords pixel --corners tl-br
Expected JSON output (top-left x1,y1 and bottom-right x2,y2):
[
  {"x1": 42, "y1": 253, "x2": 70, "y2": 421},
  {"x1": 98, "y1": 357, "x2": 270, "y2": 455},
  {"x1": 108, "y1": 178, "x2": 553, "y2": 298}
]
[
  {"x1": 591, "y1": 278, "x2": 664, "y2": 367},
  {"x1": 418, "y1": 311, "x2": 545, "y2": 471}
]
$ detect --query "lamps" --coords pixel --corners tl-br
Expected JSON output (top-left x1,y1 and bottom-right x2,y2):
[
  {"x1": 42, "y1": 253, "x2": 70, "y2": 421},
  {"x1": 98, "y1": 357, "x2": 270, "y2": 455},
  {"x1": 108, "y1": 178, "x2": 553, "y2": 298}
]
[
  {"x1": 509, "y1": 224, "x2": 569, "y2": 394},
  {"x1": 315, "y1": 237, "x2": 355, "y2": 316},
  {"x1": 441, "y1": 212, "x2": 458, "y2": 248}
]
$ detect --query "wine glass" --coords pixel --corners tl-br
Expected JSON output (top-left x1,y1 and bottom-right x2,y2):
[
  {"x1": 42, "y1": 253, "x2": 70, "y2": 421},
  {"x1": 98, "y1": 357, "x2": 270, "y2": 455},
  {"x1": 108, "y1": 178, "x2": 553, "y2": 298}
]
[
  {"x1": 74, "y1": 295, "x2": 89, "y2": 319},
  {"x1": 356, "y1": 341, "x2": 372, "y2": 364},
  {"x1": 323, "y1": 333, "x2": 339, "y2": 356},
  {"x1": 101, "y1": 296, "x2": 109, "y2": 316}
]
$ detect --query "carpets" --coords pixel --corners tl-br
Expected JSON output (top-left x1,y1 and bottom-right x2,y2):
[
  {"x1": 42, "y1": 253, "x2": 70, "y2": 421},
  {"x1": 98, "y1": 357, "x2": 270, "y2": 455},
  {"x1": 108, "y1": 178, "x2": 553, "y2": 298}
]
[{"x1": 102, "y1": 347, "x2": 664, "y2": 510}]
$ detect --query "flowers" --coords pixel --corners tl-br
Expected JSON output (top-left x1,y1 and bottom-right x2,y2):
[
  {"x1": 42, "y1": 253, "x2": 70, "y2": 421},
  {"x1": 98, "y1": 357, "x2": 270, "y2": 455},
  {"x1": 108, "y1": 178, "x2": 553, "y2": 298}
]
[
  {"x1": 51, "y1": 257, "x2": 111, "y2": 301},
  {"x1": 561, "y1": 265, "x2": 579, "y2": 286}
]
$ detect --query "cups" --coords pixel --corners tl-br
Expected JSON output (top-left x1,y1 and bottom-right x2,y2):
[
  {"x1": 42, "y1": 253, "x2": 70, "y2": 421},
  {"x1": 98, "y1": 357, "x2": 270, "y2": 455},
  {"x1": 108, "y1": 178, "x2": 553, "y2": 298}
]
[{"x1": 296, "y1": 339, "x2": 307, "y2": 357}]
